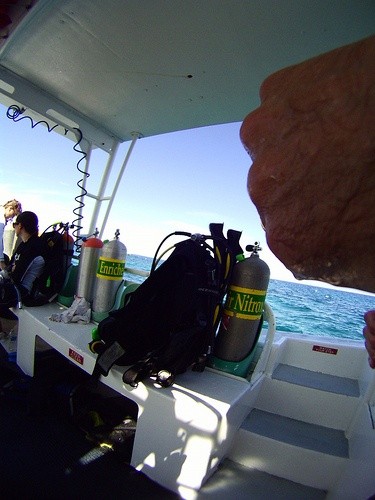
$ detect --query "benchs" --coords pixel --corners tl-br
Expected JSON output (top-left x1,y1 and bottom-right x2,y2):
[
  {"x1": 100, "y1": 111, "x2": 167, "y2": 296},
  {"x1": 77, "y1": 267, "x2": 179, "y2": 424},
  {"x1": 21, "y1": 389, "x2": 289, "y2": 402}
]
[{"x1": 8, "y1": 255, "x2": 277, "y2": 500}]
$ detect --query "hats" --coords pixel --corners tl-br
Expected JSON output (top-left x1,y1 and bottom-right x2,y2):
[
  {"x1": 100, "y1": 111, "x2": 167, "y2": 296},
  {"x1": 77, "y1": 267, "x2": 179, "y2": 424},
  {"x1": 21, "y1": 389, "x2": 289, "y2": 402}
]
[
  {"x1": 2, "y1": 199, "x2": 22, "y2": 213},
  {"x1": 16, "y1": 212, "x2": 38, "y2": 225}
]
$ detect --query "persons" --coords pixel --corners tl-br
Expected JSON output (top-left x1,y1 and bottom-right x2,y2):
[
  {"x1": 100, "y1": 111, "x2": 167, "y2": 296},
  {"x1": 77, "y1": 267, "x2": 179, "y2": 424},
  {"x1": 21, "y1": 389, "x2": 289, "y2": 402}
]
[
  {"x1": 0, "y1": 211, "x2": 48, "y2": 321},
  {"x1": 2, "y1": 200, "x2": 23, "y2": 268},
  {"x1": 240, "y1": 35, "x2": 374, "y2": 367}
]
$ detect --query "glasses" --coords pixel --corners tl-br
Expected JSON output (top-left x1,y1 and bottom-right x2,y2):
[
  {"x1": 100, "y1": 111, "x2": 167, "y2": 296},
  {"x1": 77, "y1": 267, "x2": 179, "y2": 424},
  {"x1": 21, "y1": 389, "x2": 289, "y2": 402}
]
[{"x1": 13, "y1": 221, "x2": 20, "y2": 228}]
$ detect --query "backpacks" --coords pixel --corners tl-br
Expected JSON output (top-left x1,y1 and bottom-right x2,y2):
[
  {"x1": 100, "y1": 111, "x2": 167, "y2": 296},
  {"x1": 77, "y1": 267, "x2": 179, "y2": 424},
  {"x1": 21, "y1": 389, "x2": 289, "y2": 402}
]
[
  {"x1": 88, "y1": 233, "x2": 234, "y2": 390},
  {"x1": 23, "y1": 223, "x2": 74, "y2": 308}
]
